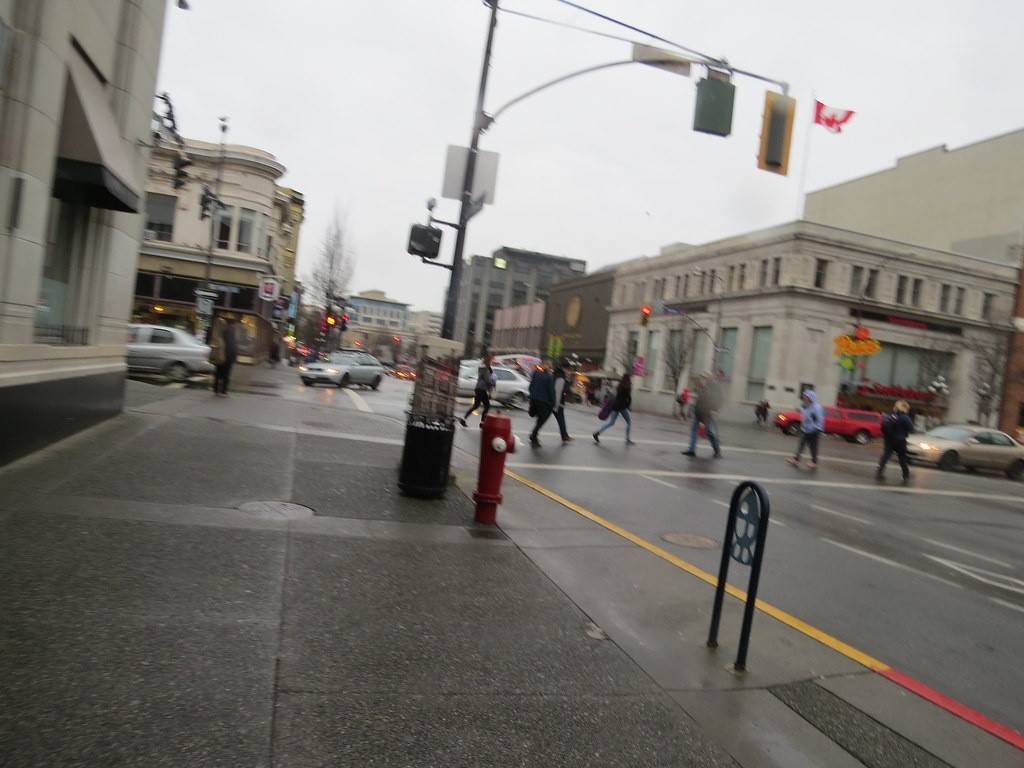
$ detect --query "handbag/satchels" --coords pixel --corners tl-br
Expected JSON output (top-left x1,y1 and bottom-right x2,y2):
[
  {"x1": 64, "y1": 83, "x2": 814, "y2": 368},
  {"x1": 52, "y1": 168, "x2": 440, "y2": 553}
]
[
  {"x1": 696, "y1": 422, "x2": 706, "y2": 439},
  {"x1": 676, "y1": 394, "x2": 685, "y2": 404},
  {"x1": 598, "y1": 397, "x2": 614, "y2": 420}
]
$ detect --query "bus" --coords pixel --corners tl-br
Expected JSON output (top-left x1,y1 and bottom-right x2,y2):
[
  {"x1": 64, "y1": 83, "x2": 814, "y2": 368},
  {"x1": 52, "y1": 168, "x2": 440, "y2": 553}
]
[{"x1": 495, "y1": 354, "x2": 541, "y2": 377}]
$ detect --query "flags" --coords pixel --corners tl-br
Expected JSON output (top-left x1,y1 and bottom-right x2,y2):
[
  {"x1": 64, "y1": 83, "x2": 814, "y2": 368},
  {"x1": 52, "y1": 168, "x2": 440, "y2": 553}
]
[{"x1": 812, "y1": 101, "x2": 855, "y2": 133}]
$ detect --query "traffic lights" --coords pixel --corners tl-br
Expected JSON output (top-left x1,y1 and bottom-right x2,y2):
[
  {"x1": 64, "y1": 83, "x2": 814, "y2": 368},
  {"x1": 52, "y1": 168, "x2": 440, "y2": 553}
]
[
  {"x1": 202, "y1": 191, "x2": 217, "y2": 221},
  {"x1": 341, "y1": 314, "x2": 347, "y2": 331},
  {"x1": 641, "y1": 304, "x2": 652, "y2": 327},
  {"x1": 178, "y1": 156, "x2": 191, "y2": 187}
]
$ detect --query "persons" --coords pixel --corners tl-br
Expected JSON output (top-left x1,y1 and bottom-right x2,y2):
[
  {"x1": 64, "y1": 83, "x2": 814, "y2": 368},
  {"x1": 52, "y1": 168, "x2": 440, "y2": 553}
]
[
  {"x1": 873, "y1": 401, "x2": 941, "y2": 483},
  {"x1": 210, "y1": 318, "x2": 317, "y2": 397},
  {"x1": 680, "y1": 371, "x2": 773, "y2": 456},
  {"x1": 785, "y1": 390, "x2": 823, "y2": 468},
  {"x1": 463, "y1": 351, "x2": 613, "y2": 446},
  {"x1": 592, "y1": 374, "x2": 635, "y2": 444}
]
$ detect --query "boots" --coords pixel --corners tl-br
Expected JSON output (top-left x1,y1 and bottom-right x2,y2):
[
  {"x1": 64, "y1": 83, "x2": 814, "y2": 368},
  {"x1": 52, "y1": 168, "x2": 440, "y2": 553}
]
[
  {"x1": 626, "y1": 436, "x2": 634, "y2": 444},
  {"x1": 593, "y1": 432, "x2": 600, "y2": 442}
]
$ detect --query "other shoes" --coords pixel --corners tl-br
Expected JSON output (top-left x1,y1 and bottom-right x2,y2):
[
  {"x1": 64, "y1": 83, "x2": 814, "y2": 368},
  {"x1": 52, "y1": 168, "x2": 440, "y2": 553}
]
[
  {"x1": 563, "y1": 436, "x2": 574, "y2": 442},
  {"x1": 875, "y1": 475, "x2": 888, "y2": 481},
  {"x1": 529, "y1": 433, "x2": 542, "y2": 447},
  {"x1": 805, "y1": 462, "x2": 820, "y2": 470},
  {"x1": 903, "y1": 474, "x2": 914, "y2": 480},
  {"x1": 460, "y1": 420, "x2": 468, "y2": 427},
  {"x1": 785, "y1": 456, "x2": 800, "y2": 467},
  {"x1": 714, "y1": 453, "x2": 722, "y2": 458},
  {"x1": 681, "y1": 451, "x2": 695, "y2": 456}
]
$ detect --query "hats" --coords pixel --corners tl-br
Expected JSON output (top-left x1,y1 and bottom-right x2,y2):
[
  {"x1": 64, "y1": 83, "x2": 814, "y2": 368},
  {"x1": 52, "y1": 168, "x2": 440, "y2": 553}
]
[
  {"x1": 895, "y1": 400, "x2": 910, "y2": 413},
  {"x1": 700, "y1": 370, "x2": 713, "y2": 380}
]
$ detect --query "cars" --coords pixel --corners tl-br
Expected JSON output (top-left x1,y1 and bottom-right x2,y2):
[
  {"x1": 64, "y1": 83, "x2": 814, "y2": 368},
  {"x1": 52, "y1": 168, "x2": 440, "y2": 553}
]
[
  {"x1": 457, "y1": 367, "x2": 531, "y2": 407},
  {"x1": 299, "y1": 350, "x2": 387, "y2": 390},
  {"x1": 775, "y1": 406, "x2": 883, "y2": 445},
  {"x1": 906, "y1": 424, "x2": 1024, "y2": 480},
  {"x1": 124, "y1": 323, "x2": 212, "y2": 381}
]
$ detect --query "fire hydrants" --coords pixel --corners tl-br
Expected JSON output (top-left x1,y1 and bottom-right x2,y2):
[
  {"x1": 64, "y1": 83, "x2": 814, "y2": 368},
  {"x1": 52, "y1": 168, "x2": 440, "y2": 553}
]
[{"x1": 473, "y1": 407, "x2": 526, "y2": 525}]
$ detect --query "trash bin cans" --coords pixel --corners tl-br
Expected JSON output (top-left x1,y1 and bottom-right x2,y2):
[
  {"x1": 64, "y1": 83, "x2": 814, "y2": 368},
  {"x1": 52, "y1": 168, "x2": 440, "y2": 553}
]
[{"x1": 397, "y1": 410, "x2": 456, "y2": 499}]
